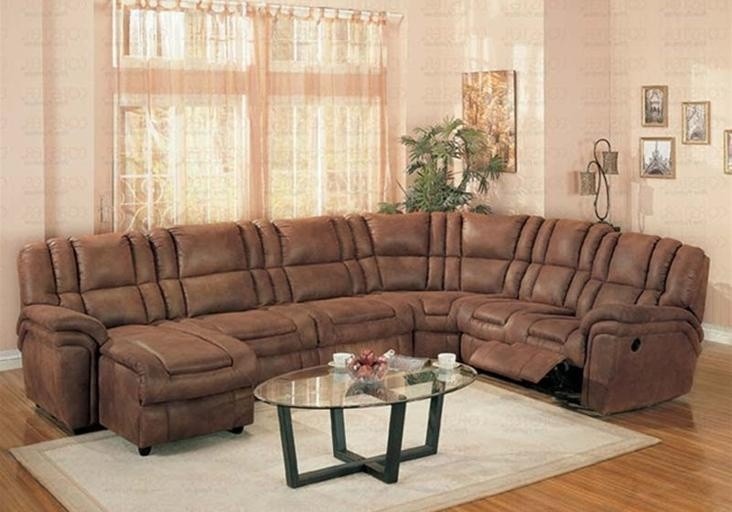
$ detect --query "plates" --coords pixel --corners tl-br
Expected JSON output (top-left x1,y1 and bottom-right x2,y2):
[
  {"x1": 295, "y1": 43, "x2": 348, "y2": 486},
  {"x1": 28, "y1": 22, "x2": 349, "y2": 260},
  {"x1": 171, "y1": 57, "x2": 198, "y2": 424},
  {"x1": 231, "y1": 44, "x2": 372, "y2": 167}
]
[
  {"x1": 433, "y1": 360, "x2": 460, "y2": 370},
  {"x1": 328, "y1": 361, "x2": 350, "y2": 368}
]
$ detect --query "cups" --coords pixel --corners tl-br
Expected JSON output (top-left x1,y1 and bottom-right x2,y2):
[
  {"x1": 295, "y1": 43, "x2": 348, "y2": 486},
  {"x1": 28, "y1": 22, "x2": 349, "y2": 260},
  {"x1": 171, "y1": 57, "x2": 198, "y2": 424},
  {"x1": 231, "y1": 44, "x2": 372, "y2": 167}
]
[
  {"x1": 332, "y1": 352, "x2": 355, "y2": 364},
  {"x1": 437, "y1": 352, "x2": 456, "y2": 366}
]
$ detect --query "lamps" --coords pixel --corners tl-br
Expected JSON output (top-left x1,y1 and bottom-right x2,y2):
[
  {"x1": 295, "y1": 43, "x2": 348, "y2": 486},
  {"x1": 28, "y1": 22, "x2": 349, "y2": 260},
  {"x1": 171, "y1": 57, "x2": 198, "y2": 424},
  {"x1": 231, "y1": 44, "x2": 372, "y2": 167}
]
[{"x1": 573, "y1": 138, "x2": 621, "y2": 230}]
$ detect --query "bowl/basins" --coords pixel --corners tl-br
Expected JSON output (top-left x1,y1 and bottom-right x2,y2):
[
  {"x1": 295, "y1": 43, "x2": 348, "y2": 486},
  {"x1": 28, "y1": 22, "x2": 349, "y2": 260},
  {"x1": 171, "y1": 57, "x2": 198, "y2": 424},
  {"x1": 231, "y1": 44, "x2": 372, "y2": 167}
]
[{"x1": 346, "y1": 363, "x2": 390, "y2": 385}]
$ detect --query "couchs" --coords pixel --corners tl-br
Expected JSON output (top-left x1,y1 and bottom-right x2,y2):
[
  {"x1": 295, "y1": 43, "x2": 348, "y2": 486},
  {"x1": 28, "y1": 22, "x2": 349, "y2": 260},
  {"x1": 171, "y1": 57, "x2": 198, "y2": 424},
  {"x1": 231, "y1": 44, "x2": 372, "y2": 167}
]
[{"x1": 16, "y1": 210, "x2": 711, "y2": 454}]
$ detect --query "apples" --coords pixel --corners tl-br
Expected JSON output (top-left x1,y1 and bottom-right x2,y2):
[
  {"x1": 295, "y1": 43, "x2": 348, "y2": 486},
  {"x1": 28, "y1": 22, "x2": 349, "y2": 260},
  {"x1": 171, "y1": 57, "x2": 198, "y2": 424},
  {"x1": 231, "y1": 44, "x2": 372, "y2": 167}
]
[{"x1": 353, "y1": 349, "x2": 388, "y2": 379}]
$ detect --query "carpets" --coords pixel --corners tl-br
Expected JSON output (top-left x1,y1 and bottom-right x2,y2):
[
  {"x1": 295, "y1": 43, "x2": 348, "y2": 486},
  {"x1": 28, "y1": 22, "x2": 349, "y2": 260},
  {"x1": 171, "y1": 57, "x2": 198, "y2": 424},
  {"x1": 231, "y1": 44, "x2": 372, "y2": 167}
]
[{"x1": 8, "y1": 365, "x2": 664, "y2": 512}]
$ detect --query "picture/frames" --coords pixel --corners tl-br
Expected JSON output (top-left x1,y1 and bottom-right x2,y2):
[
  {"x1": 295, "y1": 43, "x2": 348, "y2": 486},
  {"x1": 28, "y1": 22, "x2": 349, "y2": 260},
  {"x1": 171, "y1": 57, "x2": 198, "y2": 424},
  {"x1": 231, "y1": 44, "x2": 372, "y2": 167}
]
[
  {"x1": 721, "y1": 130, "x2": 732, "y2": 175},
  {"x1": 639, "y1": 84, "x2": 669, "y2": 128},
  {"x1": 682, "y1": 101, "x2": 711, "y2": 146},
  {"x1": 638, "y1": 136, "x2": 676, "y2": 179}
]
